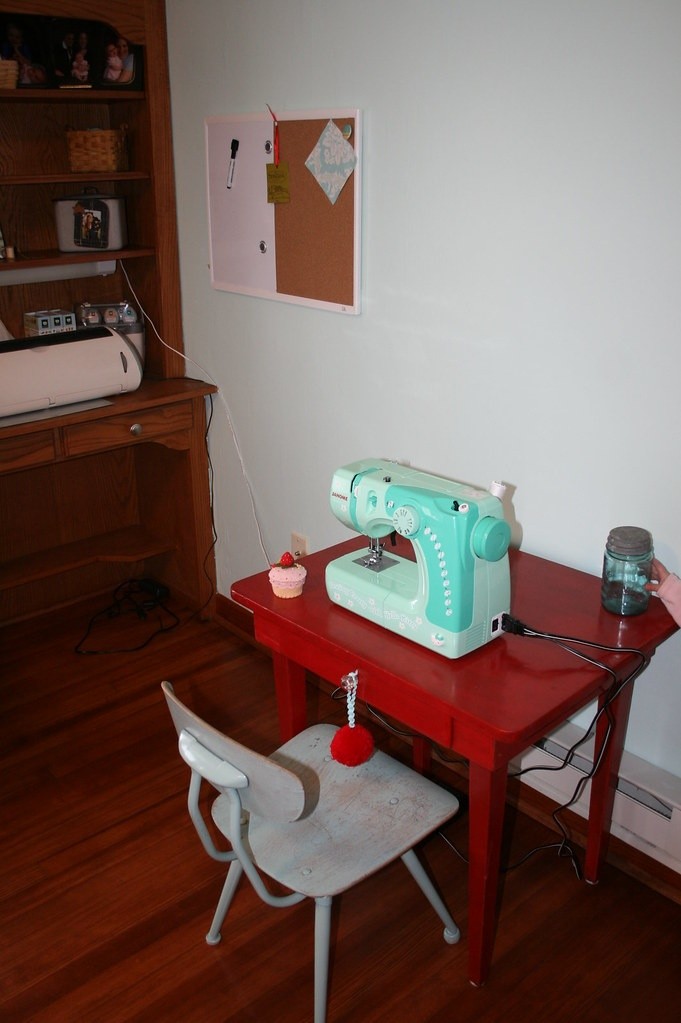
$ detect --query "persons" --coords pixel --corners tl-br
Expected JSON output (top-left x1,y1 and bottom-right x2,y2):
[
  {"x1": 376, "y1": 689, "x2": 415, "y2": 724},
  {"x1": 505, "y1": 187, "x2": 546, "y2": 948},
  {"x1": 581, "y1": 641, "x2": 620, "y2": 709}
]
[
  {"x1": 81, "y1": 213, "x2": 101, "y2": 241},
  {"x1": 638, "y1": 558, "x2": 681, "y2": 629},
  {"x1": 4, "y1": 24, "x2": 135, "y2": 85}
]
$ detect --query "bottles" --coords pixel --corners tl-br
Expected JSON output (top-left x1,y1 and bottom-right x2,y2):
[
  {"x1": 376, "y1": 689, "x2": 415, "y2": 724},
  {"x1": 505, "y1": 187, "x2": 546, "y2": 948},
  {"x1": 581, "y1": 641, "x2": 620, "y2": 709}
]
[{"x1": 600, "y1": 527, "x2": 654, "y2": 617}]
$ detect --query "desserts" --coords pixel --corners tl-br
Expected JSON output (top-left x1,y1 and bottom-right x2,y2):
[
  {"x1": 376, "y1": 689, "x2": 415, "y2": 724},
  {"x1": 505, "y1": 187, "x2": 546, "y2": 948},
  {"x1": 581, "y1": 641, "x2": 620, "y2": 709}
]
[{"x1": 268, "y1": 552, "x2": 307, "y2": 598}]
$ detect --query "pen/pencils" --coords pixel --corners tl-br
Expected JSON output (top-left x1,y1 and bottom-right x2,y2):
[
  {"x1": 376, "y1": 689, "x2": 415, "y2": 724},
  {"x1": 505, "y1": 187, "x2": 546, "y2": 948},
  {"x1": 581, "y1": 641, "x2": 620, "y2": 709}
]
[
  {"x1": 226, "y1": 139, "x2": 239, "y2": 189},
  {"x1": 275, "y1": 122, "x2": 279, "y2": 168}
]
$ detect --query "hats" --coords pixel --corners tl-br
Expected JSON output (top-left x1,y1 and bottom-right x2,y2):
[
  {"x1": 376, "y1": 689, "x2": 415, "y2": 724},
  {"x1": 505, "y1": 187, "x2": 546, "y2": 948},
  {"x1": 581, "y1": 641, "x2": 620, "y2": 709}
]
[{"x1": 93, "y1": 217, "x2": 100, "y2": 222}]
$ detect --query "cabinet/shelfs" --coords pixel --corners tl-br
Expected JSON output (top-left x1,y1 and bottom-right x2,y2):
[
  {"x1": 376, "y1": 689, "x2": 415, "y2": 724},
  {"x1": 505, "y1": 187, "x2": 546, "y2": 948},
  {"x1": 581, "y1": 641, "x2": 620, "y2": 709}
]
[{"x1": 0, "y1": 0, "x2": 219, "y2": 623}]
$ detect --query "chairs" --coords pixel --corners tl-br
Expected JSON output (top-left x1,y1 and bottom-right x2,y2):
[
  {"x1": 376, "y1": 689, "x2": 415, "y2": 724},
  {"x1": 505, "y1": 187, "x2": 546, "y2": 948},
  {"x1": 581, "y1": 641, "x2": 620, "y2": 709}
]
[{"x1": 161, "y1": 680, "x2": 459, "y2": 1023}]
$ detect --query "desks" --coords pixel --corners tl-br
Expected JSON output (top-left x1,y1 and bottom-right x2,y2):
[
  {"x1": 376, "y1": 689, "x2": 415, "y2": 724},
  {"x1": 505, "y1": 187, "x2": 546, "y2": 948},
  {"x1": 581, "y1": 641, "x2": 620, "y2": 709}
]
[{"x1": 231, "y1": 531, "x2": 680, "y2": 987}]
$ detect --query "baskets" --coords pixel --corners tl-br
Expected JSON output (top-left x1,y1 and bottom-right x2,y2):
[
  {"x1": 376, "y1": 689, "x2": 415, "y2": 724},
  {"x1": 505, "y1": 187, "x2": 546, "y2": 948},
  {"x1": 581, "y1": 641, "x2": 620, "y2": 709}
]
[
  {"x1": 0, "y1": 60, "x2": 18, "y2": 90},
  {"x1": 63, "y1": 122, "x2": 129, "y2": 173}
]
